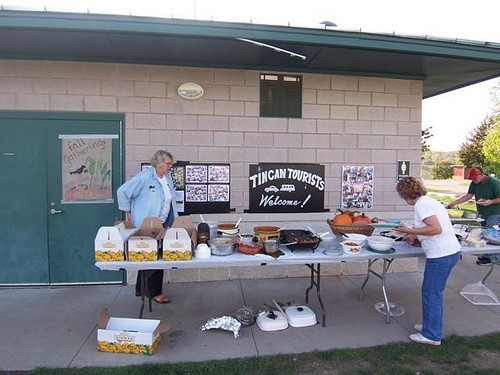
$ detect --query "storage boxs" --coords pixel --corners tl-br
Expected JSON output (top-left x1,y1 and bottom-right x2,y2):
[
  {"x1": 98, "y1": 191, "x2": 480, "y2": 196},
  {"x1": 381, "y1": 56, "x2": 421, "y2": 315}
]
[
  {"x1": 94, "y1": 221, "x2": 137, "y2": 261},
  {"x1": 97, "y1": 307, "x2": 162, "y2": 355},
  {"x1": 162, "y1": 215, "x2": 198, "y2": 260},
  {"x1": 126, "y1": 217, "x2": 168, "y2": 261}
]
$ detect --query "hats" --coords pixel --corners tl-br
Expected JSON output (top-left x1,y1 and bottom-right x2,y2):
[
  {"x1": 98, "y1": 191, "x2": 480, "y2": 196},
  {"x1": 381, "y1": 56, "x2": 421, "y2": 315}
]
[{"x1": 466, "y1": 167, "x2": 483, "y2": 180}]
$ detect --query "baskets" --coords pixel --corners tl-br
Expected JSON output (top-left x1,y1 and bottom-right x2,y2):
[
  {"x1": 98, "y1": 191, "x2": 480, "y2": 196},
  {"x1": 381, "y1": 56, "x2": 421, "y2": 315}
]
[{"x1": 327, "y1": 219, "x2": 375, "y2": 236}]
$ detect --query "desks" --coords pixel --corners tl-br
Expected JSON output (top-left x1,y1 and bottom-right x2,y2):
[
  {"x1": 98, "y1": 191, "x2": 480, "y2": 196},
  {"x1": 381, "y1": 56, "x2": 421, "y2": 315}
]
[{"x1": 95, "y1": 219, "x2": 500, "y2": 334}]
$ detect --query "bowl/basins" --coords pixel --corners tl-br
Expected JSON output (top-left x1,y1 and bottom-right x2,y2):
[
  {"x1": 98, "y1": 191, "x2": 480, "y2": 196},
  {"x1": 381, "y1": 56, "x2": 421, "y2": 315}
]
[
  {"x1": 464, "y1": 211, "x2": 479, "y2": 218},
  {"x1": 367, "y1": 236, "x2": 395, "y2": 251},
  {"x1": 340, "y1": 240, "x2": 364, "y2": 254},
  {"x1": 447, "y1": 209, "x2": 464, "y2": 218},
  {"x1": 208, "y1": 238, "x2": 235, "y2": 255},
  {"x1": 342, "y1": 233, "x2": 367, "y2": 248}
]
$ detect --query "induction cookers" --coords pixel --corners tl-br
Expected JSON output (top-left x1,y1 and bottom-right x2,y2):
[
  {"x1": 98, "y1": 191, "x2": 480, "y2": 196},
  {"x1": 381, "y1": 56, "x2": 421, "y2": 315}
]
[{"x1": 280, "y1": 230, "x2": 321, "y2": 252}]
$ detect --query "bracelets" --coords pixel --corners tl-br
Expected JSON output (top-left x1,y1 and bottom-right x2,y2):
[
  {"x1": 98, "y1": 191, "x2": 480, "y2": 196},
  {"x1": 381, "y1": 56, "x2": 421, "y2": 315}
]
[{"x1": 447, "y1": 205, "x2": 451, "y2": 209}]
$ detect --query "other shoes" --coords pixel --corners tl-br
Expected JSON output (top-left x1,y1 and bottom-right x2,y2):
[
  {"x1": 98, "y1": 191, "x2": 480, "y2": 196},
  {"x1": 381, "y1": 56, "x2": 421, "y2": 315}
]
[
  {"x1": 152, "y1": 294, "x2": 170, "y2": 304},
  {"x1": 414, "y1": 324, "x2": 423, "y2": 332},
  {"x1": 478, "y1": 256, "x2": 486, "y2": 260},
  {"x1": 476, "y1": 258, "x2": 492, "y2": 265},
  {"x1": 409, "y1": 332, "x2": 441, "y2": 345}
]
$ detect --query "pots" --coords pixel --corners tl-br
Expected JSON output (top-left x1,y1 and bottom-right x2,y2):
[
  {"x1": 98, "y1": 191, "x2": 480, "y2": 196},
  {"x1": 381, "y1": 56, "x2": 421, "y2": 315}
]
[
  {"x1": 254, "y1": 226, "x2": 280, "y2": 243},
  {"x1": 217, "y1": 223, "x2": 240, "y2": 236}
]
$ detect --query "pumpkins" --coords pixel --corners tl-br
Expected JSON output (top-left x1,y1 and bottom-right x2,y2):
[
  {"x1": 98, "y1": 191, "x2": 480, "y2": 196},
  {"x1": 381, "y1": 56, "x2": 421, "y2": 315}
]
[{"x1": 332, "y1": 209, "x2": 371, "y2": 225}]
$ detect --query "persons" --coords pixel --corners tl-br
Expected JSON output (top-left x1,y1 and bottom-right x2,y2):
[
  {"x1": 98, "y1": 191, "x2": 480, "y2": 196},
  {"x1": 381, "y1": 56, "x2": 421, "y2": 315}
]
[
  {"x1": 445, "y1": 167, "x2": 500, "y2": 265},
  {"x1": 395, "y1": 177, "x2": 462, "y2": 345},
  {"x1": 117, "y1": 150, "x2": 179, "y2": 304}
]
